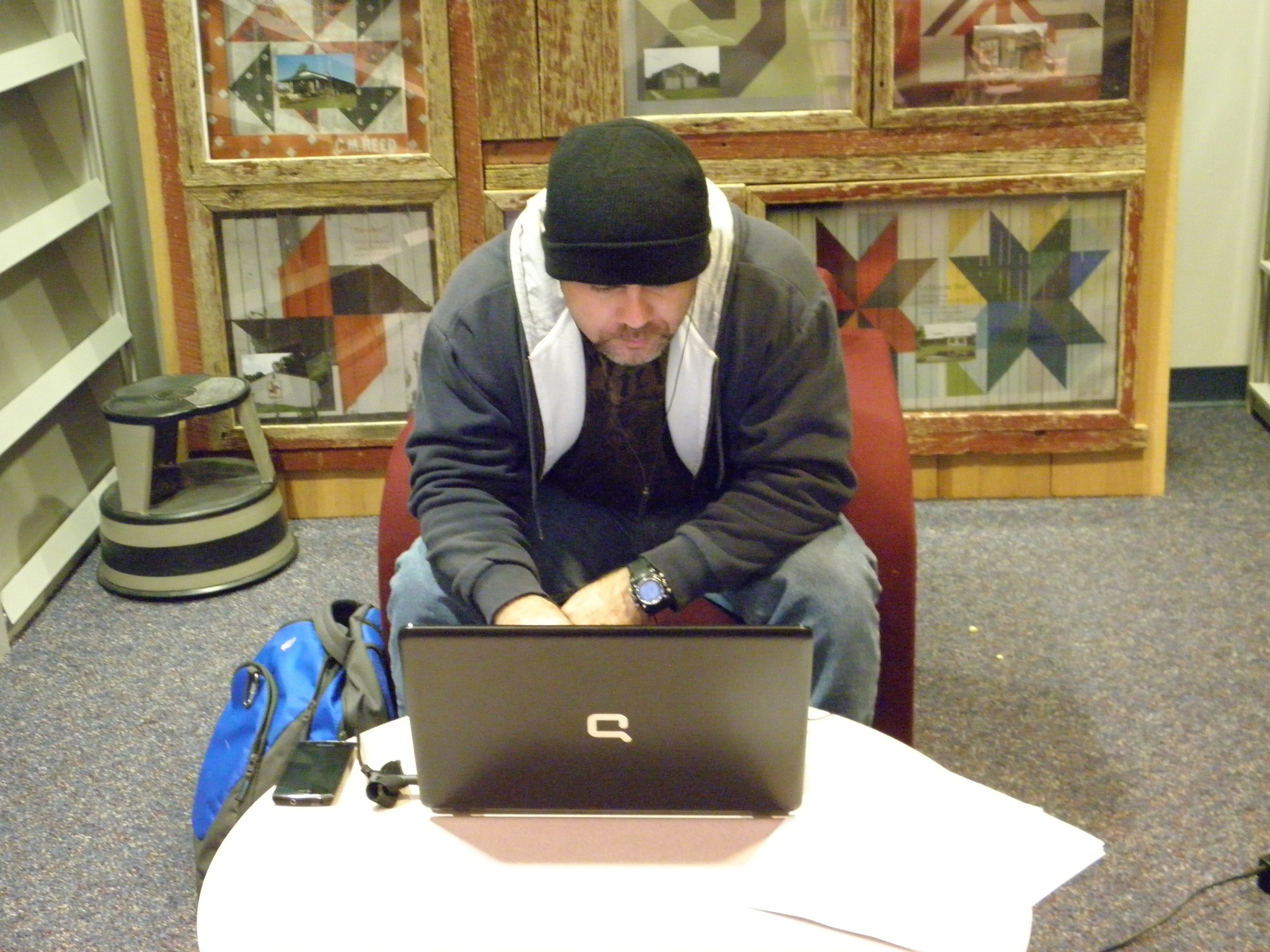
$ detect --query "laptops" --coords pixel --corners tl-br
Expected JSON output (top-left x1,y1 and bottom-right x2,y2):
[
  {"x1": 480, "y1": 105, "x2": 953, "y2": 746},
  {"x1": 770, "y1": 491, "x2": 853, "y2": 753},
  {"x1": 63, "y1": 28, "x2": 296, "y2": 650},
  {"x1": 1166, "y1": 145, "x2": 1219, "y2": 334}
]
[{"x1": 396, "y1": 625, "x2": 813, "y2": 816}]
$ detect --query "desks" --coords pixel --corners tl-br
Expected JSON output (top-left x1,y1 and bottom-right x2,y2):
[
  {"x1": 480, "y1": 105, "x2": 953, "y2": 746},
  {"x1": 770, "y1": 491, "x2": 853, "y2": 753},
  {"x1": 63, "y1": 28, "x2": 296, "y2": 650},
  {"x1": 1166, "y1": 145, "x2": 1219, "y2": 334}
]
[{"x1": 196, "y1": 715, "x2": 1035, "y2": 951}]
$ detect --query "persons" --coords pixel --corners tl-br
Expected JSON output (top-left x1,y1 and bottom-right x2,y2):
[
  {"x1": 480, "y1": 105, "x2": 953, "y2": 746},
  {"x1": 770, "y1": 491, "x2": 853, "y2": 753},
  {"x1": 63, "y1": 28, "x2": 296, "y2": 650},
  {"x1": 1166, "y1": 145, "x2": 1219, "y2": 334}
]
[{"x1": 386, "y1": 117, "x2": 880, "y2": 727}]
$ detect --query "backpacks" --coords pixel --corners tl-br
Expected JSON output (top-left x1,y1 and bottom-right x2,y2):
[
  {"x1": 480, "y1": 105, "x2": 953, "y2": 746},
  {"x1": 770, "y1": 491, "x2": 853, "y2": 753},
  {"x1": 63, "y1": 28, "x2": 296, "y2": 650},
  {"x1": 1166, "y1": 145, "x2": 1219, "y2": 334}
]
[{"x1": 191, "y1": 599, "x2": 397, "y2": 900}]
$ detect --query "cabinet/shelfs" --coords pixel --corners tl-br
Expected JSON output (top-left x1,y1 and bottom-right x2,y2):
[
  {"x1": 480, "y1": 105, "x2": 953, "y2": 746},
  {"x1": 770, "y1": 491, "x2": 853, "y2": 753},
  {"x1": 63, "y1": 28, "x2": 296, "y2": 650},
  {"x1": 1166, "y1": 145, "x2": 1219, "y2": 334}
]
[{"x1": 1245, "y1": 159, "x2": 1270, "y2": 428}]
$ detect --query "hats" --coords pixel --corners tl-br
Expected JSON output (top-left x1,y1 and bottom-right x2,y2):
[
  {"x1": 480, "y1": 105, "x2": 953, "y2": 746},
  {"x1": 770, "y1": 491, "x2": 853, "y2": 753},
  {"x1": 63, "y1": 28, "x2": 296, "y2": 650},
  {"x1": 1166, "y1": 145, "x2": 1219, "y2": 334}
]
[{"x1": 541, "y1": 118, "x2": 711, "y2": 285}]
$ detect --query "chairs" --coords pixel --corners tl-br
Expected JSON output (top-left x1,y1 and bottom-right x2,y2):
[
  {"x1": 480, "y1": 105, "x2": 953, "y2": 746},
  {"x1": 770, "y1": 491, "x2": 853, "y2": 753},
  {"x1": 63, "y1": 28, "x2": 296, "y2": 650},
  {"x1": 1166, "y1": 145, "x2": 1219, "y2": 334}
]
[{"x1": 378, "y1": 328, "x2": 917, "y2": 746}]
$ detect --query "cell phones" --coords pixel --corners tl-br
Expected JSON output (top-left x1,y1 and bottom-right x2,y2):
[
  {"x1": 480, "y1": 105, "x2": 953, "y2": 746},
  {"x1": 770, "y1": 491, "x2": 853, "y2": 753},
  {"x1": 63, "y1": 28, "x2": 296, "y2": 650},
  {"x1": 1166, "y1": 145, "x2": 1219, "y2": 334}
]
[{"x1": 273, "y1": 742, "x2": 357, "y2": 806}]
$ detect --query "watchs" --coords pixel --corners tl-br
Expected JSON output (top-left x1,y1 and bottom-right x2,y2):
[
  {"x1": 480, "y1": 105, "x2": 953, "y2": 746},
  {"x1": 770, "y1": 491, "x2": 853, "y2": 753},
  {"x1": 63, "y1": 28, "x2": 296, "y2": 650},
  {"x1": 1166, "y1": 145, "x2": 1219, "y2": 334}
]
[{"x1": 626, "y1": 558, "x2": 671, "y2": 616}]
